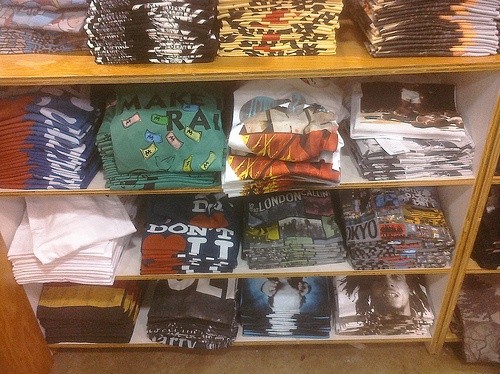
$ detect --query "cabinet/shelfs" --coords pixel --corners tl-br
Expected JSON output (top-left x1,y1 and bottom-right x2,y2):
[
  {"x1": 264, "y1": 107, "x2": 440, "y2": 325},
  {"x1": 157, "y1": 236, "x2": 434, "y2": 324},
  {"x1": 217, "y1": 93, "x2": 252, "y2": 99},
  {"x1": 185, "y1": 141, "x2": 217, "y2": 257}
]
[{"x1": 0, "y1": 0, "x2": 500, "y2": 356}]
